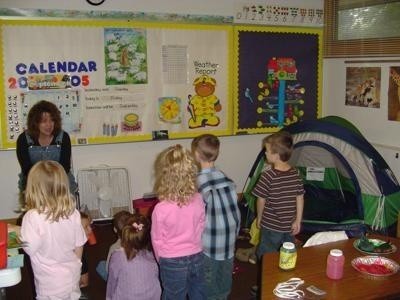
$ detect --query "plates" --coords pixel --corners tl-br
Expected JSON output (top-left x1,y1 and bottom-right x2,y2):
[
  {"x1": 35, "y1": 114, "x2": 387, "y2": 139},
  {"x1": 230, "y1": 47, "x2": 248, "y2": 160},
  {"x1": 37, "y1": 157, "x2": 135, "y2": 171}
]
[
  {"x1": 352, "y1": 238, "x2": 397, "y2": 255},
  {"x1": 351, "y1": 255, "x2": 399, "y2": 277}
]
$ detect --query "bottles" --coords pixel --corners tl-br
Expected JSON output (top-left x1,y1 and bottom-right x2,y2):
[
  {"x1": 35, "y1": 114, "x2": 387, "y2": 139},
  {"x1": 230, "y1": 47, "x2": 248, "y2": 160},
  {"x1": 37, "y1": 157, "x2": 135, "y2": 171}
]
[
  {"x1": 278, "y1": 242, "x2": 297, "y2": 271},
  {"x1": 326, "y1": 249, "x2": 345, "y2": 280}
]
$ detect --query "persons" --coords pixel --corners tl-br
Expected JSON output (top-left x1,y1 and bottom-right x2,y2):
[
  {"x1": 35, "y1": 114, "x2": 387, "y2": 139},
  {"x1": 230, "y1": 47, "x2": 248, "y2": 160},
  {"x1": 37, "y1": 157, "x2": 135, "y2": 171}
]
[
  {"x1": 251, "y1": 131, "x2": 307, "y2": 296},
  {"x1": 150, "y1": 144, "x2": 208, "y2": 300},
  {"x1": 191, "y1": 133, "x2": 241, "y2": 300},
  {"x1": 19, "y1": 160, "x2": 87, "y2": 300},
  {"x1": 14, "y1": 100, "x2": 79, "y2": 209},
  {"x1": 96, "y1": 209, "x2": 133, "y2": 281},
  {"x1": 106, "y1": 214, "x2": 162, "y2": 300},
  {"x1": 79, "y1": 211, "x2": 96, "y2": 288}
]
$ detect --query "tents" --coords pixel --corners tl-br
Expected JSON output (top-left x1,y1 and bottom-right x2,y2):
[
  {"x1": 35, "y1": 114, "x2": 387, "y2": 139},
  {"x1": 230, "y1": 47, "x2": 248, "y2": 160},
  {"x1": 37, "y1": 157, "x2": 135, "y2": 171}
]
[{"x1": 237, "y1": 115, "x2": 400, "y2": 238}]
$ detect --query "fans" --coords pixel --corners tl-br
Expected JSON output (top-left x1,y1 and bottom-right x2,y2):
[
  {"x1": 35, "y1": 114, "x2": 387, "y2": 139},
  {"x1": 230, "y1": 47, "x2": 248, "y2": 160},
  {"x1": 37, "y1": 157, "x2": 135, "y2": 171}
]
[{"x1": 77, "y1": 166, "x2": 134, "y2": 221}]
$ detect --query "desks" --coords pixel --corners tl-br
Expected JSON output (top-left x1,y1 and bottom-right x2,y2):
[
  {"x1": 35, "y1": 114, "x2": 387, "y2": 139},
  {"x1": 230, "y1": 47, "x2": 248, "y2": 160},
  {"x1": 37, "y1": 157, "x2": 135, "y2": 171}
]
[{"x1": 262, "y1": 234, "x2": 400, "y2": 300}]
